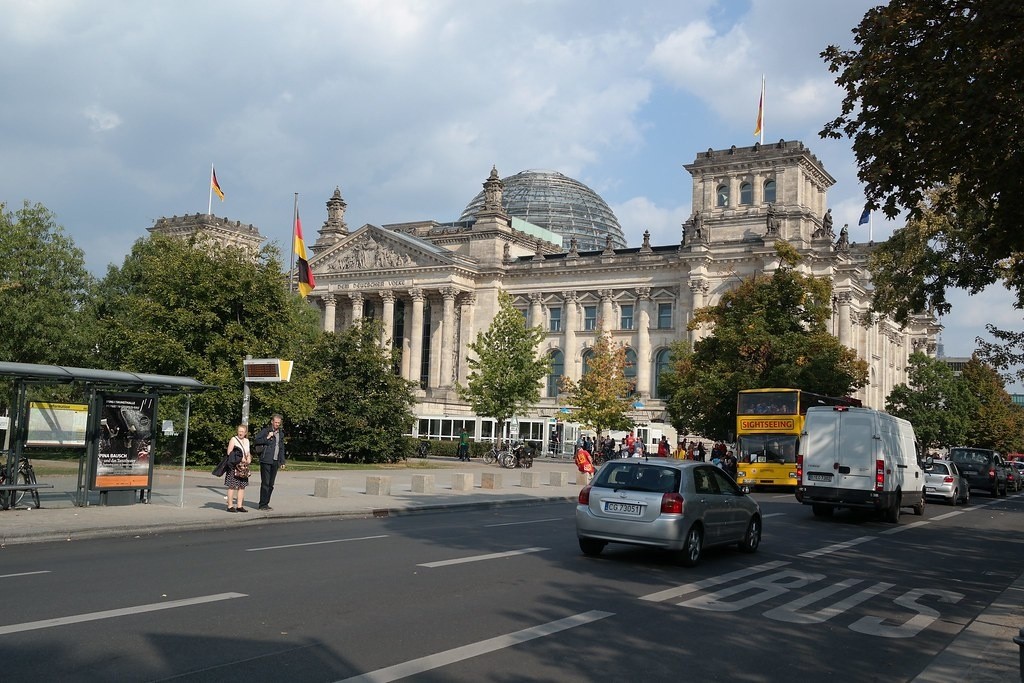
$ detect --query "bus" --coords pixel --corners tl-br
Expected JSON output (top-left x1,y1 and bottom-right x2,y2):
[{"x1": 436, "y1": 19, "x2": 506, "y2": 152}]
[{"x1": 733, "y1": 387, "x2": 863, "y2": 493}]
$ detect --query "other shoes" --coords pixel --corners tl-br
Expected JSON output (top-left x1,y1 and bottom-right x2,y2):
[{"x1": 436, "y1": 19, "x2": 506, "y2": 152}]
[
  {"x1": 259, "y1": 505, "x2": 273, "y2": 512},
  {"x1": 227, "y1": 506, "x2": 236, "y2": 512},
  {"x1": 236, "y1": 507, "x2": 248, "y2": 512}
]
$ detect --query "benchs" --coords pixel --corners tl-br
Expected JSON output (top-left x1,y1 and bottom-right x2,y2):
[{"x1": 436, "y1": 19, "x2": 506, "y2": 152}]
[{"x1": 0, "y1": 482, "x2": 55, "y2": 508}]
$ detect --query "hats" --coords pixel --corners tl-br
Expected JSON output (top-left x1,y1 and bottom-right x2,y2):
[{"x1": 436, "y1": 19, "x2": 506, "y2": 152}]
[{"x1": 576, "y1": 445, "x2": 581, "y2": 451}]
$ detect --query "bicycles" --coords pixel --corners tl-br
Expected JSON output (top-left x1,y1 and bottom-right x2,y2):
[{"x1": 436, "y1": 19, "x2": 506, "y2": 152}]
[
  {"x1": 457, "y1": 442, "x2": 471, "y2": 462},
  {"x1": 0, "y1": 444, "x2": 41, "y2": 509},
  {"x1": 482, "y1": 440, "x2": 540, "y2": 469}
]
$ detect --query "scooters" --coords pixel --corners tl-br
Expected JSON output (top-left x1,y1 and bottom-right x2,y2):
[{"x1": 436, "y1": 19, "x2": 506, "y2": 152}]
[
  {"x1": 590, "y1": 447, "x2": 622, "y2": 466},
  {"x1": 414, "y1": 439, "x2": 433, "y2": 458}
]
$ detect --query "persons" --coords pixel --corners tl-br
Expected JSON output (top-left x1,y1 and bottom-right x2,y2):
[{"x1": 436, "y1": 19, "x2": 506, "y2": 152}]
[
  {"x1": 225, "y1": 425, "x2": 251, "y2": 512},
  {"x1": 927, "y1": 451, "x2": 950, "y2": 460},
  {"x1": 570, "y1": 237, "x2": 577, "y2": 249},
  {"x1": 643, "y1": 230, "x2": 650, "y2": 245},
  {"x1": 575, "y1": 447, "x2": 597, "y2": 475},
  {"x1": 824, "y1": 208, "x2": 833, "y2": 235},
  {"x1": 749, "y1": 403, "x2": 787, "y2": 413},
  {"x1": 694, "y1": 210, "x2": 704, "y2": 239},
  {"x1": 659, "y1": 436, "x2": 737, "y2": 479},
  {"x1": 504, "y1": 242, "x2": 510, "y2": 254},
  {"x1": 537, "y1": 239, "x2": 542, "y2": 251},
  {"x1": 332, "y1": 243, "x2": 411, "y2": 270},
  {"x1": 606, "y1": 234, "x2": 613, "y2": 248},
  {"x1": 254, "y1": 414, "x2": 286, "y2": 511},
  {"x1": 459, "y1": 428, "x2": 470, "y2": 461},
  {"x1": 551, "y1": 431, "x2": 560, "y2": 457},
  {"x1": 767, "y1": 202, "x2": 775, "y2": 231},
  {"x1": 577, "y1": 431, "x2": 646, "y2": 459},
  {"x1": 840, "y1": 223, "x2": 848, "y2": 244}
]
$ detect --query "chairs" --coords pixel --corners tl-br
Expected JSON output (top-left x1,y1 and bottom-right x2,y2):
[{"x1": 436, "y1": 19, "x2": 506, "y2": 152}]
[
  {"x1": 638, "y1": 469, "x2": 660, "y2": 488},
  {"x1": 659, "y1": 475, "x2": 676, "y2": 489},
  {"x1": 614, "y1": 469, "x2": 631, "y2": 485},
  {"x1": 965, "y1": 453, "x2": 973, "y2": 462}
]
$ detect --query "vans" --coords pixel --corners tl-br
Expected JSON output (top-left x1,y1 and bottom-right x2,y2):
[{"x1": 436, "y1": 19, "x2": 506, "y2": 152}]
[{"x1": 796, "y1": 405, "x2": 933, "y2": 525}]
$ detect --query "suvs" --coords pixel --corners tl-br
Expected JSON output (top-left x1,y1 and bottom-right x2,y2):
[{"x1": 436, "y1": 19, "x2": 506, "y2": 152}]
[{"x1": 947, "y1": 447, "x2": 1010, "y2": 499}]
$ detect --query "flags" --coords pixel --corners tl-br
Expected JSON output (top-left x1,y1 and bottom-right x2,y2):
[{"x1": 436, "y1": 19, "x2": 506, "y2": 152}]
[
  {"x1": 295, "y1": 209, "x2": 315, "y2": 298},
  {"x1": 859, "y1": 208, "x2": 870, "y2": 225},
  {"x1": 754, "y1": 92, "x2": 763, "y2": 134},
  {"x1": 212, "y1": 170, "x2": 224, "y2": 201}
]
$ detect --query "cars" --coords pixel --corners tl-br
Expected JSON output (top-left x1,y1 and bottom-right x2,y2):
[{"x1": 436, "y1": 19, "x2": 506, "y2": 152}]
[
  {"x1": 920, "y1": 458, "x2": 971, "y2": 506},
  {"x1": 575, "y1": 455, "x2": 764, "y2": 567},
  {"x1": 1003, "y1": 452, "x2": 1024, "y2": 493}
]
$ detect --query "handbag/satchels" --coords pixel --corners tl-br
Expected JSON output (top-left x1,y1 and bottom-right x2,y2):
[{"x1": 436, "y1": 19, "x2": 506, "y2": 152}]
[
  {"x1": 234, "y1": 461, "x2": 251, "y2": 479},
  {"x1": 254, "y1": 428, "x2": 271, "y2": 456}
]
